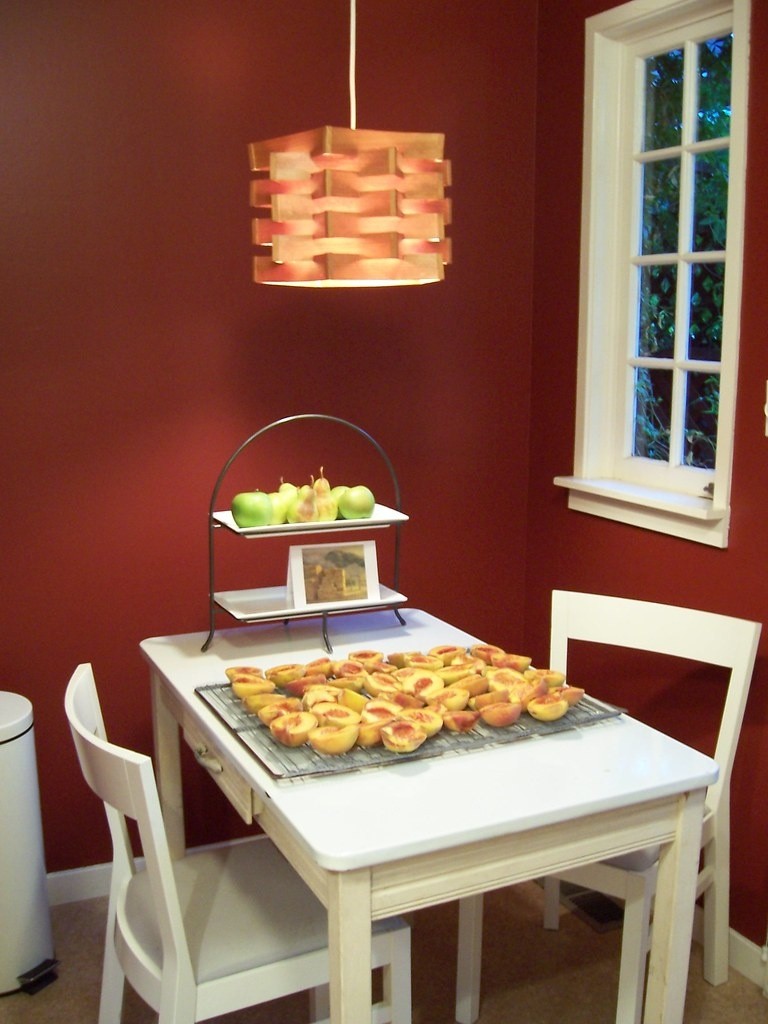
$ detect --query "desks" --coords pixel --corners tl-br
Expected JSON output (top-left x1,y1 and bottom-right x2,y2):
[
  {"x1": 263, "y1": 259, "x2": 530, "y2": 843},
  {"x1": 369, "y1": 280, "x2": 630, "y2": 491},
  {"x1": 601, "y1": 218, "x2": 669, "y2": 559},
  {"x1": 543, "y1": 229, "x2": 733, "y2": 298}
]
[{"x1": 140, "y1": 609, "x2": 720, "y2": 1024}]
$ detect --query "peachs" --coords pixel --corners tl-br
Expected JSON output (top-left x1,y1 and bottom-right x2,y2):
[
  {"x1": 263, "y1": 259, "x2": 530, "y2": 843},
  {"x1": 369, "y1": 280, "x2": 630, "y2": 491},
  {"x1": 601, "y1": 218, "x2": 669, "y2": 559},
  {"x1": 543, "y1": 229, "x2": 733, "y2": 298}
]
[{"x1": 225, "y1": 646, "x2": 585, "y2": 755}]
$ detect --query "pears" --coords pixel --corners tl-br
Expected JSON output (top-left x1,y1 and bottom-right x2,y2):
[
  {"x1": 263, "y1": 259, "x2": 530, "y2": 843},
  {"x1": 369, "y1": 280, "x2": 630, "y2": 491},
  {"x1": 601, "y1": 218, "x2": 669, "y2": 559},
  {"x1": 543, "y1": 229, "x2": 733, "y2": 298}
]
[{"x1": 266, "y1": 479, "x2": 338, "y2": 524}]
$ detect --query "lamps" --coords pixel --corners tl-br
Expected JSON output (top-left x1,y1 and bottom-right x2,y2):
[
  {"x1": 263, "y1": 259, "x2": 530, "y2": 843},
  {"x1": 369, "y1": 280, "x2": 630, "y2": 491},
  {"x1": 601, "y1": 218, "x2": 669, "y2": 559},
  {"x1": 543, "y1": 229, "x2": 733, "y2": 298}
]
[{"x1": 248, "y1": 0, "x2": 451, "y2": 288}]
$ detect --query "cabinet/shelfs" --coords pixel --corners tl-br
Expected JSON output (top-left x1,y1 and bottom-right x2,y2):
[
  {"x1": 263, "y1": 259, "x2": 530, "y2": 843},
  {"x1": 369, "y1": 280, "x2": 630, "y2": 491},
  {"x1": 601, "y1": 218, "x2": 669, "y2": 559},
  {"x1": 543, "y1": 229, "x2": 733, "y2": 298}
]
[{"x1": 200, "y1": 415, "x2": 406, "y2": 654}]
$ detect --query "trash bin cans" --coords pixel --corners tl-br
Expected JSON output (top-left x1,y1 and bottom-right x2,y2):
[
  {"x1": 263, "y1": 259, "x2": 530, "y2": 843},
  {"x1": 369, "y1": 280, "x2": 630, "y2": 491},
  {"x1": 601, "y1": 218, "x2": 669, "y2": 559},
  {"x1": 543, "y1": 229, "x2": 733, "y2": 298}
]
[{"x1": 0, "y1": 690, "x2": 61, "y2": 996}]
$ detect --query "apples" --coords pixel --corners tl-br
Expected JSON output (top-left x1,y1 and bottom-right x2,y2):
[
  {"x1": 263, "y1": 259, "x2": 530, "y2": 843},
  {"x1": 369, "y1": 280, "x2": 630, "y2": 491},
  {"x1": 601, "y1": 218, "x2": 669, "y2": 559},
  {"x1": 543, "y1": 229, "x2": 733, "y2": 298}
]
[{"x1": 232, "y1": 486, "x2": 375, "y2": 528}]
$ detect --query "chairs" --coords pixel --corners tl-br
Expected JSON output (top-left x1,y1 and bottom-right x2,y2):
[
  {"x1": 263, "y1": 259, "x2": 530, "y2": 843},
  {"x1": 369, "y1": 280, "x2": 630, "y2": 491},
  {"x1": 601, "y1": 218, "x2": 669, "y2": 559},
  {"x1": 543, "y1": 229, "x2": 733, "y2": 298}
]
[
  {"x1": 64, "y1": 662, "x2": 412, "y2": 1024},
  {"x1": 455, "y1": 589, "x2": 762, "y2": 1024}
]
